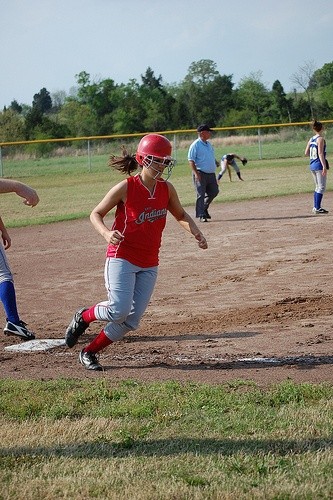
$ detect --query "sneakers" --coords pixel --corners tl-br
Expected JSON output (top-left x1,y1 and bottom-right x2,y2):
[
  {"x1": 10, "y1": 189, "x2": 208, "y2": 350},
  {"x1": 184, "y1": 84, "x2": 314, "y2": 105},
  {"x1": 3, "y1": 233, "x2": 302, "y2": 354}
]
[
  {"x1": 78, "y1": 348, "x2": 102, "y2": 371},
  {"x1": 3, "y1": 318, "x2": 34, "y2": 341},
  {"x1": 64, "y1": 307, "x2": 89, "y2": 348},
  {"x1": 312, "y1": 207, "x2": 328, "y2": 214},
  {"x1": 199, "y1": 215, "x2": 211, "y2": 222}
]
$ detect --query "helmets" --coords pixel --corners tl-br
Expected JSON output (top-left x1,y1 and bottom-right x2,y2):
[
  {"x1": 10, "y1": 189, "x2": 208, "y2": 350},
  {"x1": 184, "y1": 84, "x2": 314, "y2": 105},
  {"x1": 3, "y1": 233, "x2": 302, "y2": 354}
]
[{"x1": 135, "y1": 134, "x2": 171, "y2": 165}]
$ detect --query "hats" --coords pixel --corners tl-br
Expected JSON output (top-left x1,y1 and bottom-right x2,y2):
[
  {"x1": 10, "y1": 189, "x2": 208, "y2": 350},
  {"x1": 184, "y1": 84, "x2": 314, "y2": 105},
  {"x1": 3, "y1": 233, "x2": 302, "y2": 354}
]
[{"x1": 197, "y1": 124, "x2": 213, "y2": 132}]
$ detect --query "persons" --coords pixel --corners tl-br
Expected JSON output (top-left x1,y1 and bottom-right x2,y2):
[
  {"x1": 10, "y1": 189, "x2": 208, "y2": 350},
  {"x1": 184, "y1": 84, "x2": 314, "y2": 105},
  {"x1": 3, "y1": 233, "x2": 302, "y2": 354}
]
[
  {"x1": 217, "y1": 153, "x2": 247, "y2": 185},
  {"x1": 0, "y1": 178, "x2": 40, "y2": 340},
  {"x1": 188, "y1": 124, "x2": 219, "y2": 222},
  {"x1": 305, "y1": 119, "x2": 329, "y2": 214},
  {"x1": 65, "y1": 133, "x2": 208, "y2": 370}
]
